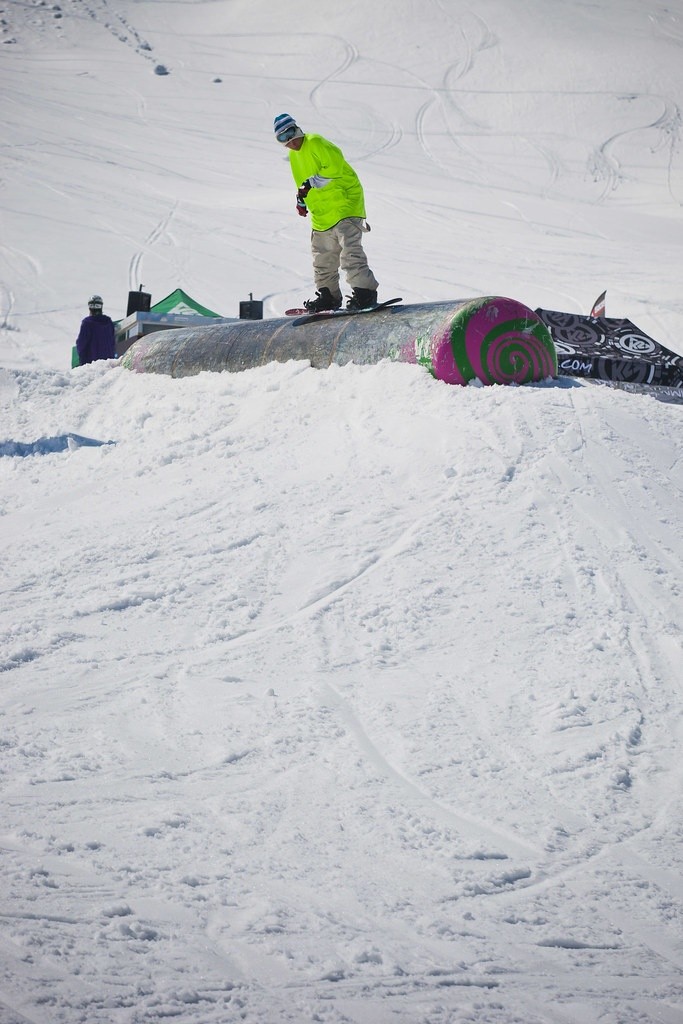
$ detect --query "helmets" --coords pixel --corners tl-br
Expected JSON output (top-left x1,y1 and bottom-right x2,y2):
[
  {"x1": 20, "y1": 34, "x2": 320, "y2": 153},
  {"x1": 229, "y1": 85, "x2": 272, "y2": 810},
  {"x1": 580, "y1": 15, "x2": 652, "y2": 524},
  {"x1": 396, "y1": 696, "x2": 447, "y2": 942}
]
[{"x1": 88, "y1": 295, "x2": 103, "y2": 310}]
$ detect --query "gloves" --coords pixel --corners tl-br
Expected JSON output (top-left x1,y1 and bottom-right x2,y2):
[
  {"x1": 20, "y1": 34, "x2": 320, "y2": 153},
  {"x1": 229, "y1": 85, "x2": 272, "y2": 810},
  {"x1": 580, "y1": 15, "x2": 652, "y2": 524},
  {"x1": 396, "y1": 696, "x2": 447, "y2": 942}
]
[
  {"x1": 296, "y1": 193, "x2": 308, "y2": 217},
  {"x1": 298, "y1": 179, "x2": 312, "y2": 198}
]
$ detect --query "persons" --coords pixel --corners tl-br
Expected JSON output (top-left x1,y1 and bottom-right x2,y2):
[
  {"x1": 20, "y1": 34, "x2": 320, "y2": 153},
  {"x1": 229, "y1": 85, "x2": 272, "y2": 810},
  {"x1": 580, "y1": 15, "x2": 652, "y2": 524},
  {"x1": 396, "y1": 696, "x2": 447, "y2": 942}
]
[
  {"x1": 77, "y1": 295, "x2": 116, "y2": 364},
  {"x1": 273, "y1": 113, "x2": 380, "y2": 312}
]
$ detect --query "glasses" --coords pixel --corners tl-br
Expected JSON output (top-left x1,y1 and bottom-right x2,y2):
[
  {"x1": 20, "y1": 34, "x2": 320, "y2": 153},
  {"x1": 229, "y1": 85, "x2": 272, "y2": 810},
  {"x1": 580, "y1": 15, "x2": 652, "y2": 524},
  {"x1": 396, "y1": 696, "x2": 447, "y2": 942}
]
[{"x1": 277, "y1": 126, "x2": 296, "y2": 143}]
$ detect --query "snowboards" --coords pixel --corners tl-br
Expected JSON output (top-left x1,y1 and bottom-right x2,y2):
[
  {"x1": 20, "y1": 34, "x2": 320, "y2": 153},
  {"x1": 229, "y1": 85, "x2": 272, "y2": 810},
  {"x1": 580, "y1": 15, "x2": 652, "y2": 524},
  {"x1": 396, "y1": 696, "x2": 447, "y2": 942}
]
[{"x1": 285, "y1": 297, "x2": 403, "y2": 317}]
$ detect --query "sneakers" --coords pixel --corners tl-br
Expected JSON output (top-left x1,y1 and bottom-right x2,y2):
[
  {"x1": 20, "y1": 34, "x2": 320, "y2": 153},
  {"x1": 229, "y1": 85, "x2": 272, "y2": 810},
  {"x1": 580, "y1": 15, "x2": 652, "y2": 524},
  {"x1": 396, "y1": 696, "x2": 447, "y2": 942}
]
[
  {"x1": 343, "y1": 287, "x2": 377, "y2": 311},
  {"x1": 303, "y1": 286, "x2": 342, "y2": 312}
]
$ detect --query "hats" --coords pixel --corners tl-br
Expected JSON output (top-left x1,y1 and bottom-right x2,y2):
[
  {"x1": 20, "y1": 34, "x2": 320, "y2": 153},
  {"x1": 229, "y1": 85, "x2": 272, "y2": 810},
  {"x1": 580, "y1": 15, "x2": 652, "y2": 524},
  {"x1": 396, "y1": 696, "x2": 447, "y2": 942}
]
[{"x1": 273, "y1": 113, "x2": 296, "y2": 137}]
